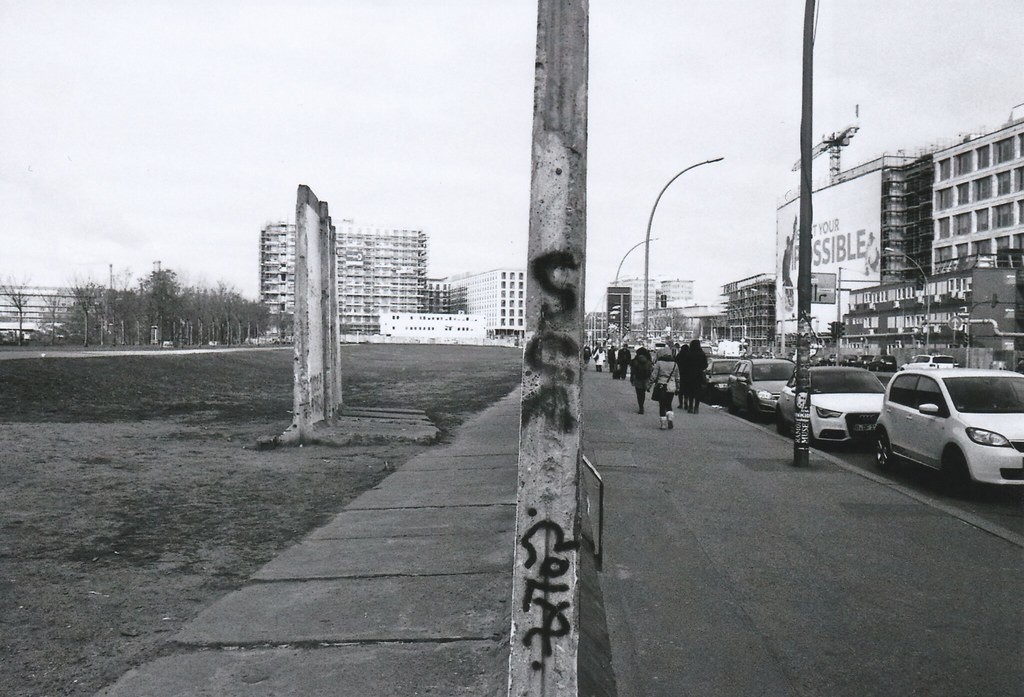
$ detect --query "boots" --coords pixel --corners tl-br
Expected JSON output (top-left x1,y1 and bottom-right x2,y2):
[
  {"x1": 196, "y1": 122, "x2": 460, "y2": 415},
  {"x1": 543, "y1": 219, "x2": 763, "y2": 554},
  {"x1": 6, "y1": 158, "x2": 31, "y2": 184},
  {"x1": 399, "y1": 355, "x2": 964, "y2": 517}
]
[
  {"x1": 660, "y1": 417, "x2": 667, "y2": 429},
  {"x1": 666, "y1": 411, "x2": 673, "y2": 428}
]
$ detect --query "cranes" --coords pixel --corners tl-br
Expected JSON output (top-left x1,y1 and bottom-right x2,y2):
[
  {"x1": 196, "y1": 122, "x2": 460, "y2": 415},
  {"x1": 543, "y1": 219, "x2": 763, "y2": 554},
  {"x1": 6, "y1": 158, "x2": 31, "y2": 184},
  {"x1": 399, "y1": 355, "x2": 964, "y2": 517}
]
[{"x1": 792, "y1": 127, "x2": 860, "y2": 178}]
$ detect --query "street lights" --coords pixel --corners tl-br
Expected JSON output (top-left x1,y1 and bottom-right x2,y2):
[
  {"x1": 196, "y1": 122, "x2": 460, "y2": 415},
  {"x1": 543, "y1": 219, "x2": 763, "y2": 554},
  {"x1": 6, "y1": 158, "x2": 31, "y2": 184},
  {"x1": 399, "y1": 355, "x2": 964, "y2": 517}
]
[
  {"x1": 643, "y1": 155, "x2": 725, "y2": 345},
  {"x1": 885, "y1": 247, "x2": 929, "y2": 354},
  {"x1": 614, "y1": 238, "x2": 657, "y2": 287}
]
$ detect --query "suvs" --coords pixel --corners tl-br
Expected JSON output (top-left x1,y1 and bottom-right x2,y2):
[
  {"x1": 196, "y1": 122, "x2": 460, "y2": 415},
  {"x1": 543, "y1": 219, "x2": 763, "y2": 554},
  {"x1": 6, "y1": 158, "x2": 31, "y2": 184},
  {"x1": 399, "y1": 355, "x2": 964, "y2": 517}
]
[{"x1": 725, "y1": 359, "x2": 795, "y2": 422}]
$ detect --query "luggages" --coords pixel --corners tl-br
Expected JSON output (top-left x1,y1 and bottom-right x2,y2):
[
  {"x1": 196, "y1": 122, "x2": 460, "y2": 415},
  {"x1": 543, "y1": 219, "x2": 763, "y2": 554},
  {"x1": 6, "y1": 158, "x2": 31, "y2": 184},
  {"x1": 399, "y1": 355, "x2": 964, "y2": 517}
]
[{"x1": 613, "y1": 363, "x2": 621, "y2": 379}]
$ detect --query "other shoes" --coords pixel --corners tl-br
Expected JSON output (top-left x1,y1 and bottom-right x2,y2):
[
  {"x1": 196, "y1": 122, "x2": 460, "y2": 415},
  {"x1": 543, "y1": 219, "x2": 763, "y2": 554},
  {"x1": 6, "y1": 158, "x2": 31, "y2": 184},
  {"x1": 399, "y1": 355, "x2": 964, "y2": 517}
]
[
  {"x1": 685, "y1": 406, "x2": 688, "y2": 410},
  {"x1": 638, "y1": 410, "x2": 644, "y2": 414},
  {"x1": 694, "y1": 409, "x2": 700, "y2": 414},
  {"x1": 677, "y1": 405, "x2": 683, "y2": 408},
  {"x1": 688, "y1": 409, "x2": 693, "y2": 413}
]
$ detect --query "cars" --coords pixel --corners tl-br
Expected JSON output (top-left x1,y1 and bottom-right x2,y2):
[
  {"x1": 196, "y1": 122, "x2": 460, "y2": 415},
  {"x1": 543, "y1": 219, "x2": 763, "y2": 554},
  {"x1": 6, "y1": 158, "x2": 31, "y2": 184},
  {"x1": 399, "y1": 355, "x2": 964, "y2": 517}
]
[
  {"x1": 900, "y1": 355, "x2": 958, "y2": 371},
  {"x1": 703, "y1": 358, "x2": 740, "y2": 407},
  {"x1": 775, "y1": 367, "x2": 886, "y2": 449},
  {"x1": 874, "y1": 367, "x2": 1024, "y2": 498},
  {"x1": 749, "y1": 349, "x2": 897, "y2": 373}
]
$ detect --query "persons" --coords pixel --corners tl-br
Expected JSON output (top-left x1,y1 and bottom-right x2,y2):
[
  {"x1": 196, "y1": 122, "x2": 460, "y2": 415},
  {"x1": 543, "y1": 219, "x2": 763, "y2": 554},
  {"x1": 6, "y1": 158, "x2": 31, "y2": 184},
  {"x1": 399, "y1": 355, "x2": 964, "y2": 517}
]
[
  {"x1": 618, "y1": 344, "x2": 631, "y2": 380},
  {"x1": 684, "y1": 339, "x2": 708, "y2": 414},
  {"x1": 630, "y1": 347, "x2": 654, "y2": 415},
  {"x1": 676, "y1": 344, "x2": 689, "y2": 410},
  {"x1": 646, "y1": 347, "x2": 680, "y2": 430},
  {"x1": 582, "y1": 345, "x2": 618, "y2": 373}
]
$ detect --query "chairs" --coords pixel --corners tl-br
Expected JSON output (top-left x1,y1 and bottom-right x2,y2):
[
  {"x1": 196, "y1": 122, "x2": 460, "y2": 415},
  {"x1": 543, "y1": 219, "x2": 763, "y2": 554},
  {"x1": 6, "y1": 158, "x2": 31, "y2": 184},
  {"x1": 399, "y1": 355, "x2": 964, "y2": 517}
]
[
  {"x1": 753, "y1": 367, "x2": 762, "y2": 378},
  {"x1": 716, "y1": 366, "x2": 730, "y2": 374}
]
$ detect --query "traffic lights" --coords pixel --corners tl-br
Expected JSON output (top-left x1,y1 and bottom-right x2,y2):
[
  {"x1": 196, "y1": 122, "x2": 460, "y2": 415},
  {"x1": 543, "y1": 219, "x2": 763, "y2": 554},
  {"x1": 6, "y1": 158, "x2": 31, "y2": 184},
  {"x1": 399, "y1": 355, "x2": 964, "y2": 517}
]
[
  {"x1": 838, "y1": 321, "x2": 845, "y2": 338},
  {"x1": 827, "y1": 321, "x2": 836, "y2": 339}
]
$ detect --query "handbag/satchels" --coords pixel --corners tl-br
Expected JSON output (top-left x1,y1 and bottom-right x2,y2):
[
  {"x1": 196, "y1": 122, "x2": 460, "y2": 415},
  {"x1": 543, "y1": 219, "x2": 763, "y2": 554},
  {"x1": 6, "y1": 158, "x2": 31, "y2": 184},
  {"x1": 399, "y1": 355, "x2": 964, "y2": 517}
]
[
  {"x1": 594, "y1": 354, "x2": 599, "y2": 361},
  {"x1": 651, "y1": 383, "x2": 667, "y2": 400}
]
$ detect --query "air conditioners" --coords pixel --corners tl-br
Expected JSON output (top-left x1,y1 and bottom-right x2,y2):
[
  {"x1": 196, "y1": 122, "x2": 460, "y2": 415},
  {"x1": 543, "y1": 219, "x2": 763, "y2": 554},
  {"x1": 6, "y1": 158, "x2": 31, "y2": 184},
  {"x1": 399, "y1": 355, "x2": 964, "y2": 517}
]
[{"x1": 847, "y1": 291, "x2": 964, "y2": 335}]
